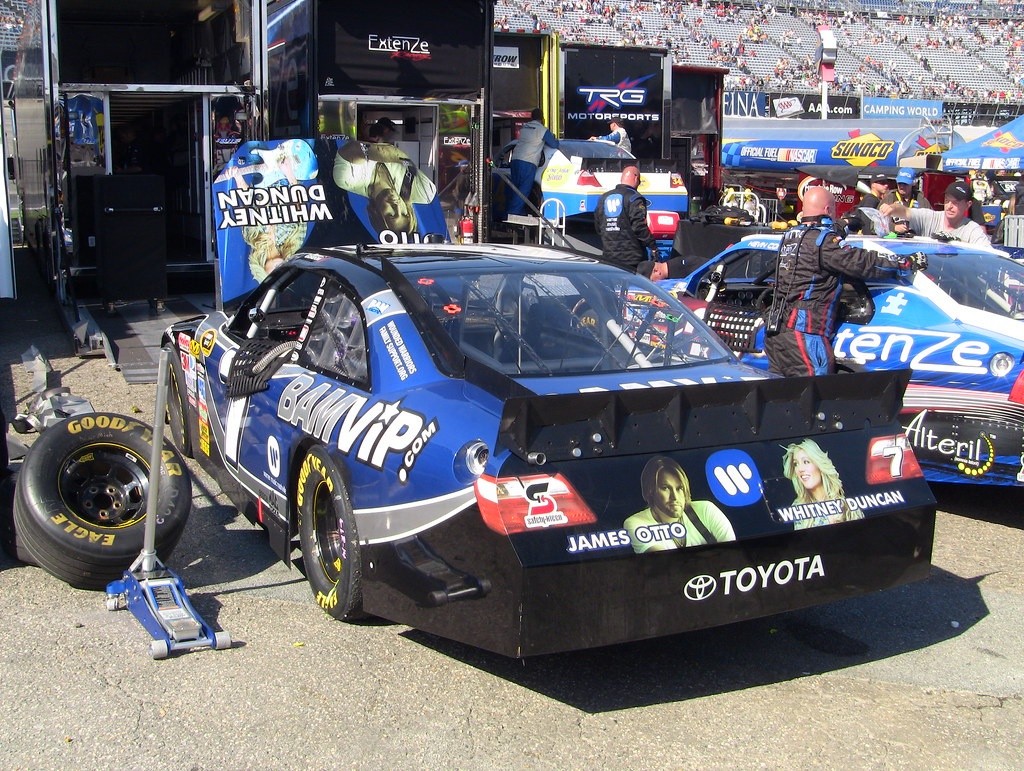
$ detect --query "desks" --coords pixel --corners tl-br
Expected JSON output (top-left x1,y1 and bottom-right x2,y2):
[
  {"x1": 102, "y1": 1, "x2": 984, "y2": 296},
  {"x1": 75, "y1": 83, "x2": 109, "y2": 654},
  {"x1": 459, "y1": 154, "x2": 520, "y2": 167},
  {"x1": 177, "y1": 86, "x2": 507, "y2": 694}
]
[{"x1": 672, "y1": 220, "x2": 772, "y2": 256}]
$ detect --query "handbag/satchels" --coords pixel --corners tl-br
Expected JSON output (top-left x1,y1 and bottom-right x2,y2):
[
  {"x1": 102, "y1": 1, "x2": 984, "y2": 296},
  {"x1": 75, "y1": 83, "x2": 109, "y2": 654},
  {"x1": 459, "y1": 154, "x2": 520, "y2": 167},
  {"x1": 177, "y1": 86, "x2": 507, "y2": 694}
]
[{"x1": 991, "y1": 195, "x2": 1016, "y2": 244}]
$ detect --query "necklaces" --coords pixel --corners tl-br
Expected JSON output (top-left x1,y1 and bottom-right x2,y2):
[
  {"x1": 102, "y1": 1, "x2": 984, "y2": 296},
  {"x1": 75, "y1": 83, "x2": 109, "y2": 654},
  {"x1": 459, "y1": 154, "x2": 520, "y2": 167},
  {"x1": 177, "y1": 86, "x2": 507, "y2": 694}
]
[
  {"x1": 371, "y1": 164, "x2": 394, "y2": 196},
  {"x1": 674, "y1": 519, "x2": 684, "y2": 547}
]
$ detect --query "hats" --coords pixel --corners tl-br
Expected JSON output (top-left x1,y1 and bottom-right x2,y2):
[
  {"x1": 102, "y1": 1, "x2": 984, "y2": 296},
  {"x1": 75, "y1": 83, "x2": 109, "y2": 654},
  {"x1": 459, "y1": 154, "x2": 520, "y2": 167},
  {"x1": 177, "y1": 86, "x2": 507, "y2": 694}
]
[
  {"x1": 942, "y1": 181, "x2": 972, "y2": 201},
  {"x1": 870, "y1": 172, "x2": 891, "y2": 184},
  {"x1": 895, "y1": 167, "x2": 916, "y2": 185},
  {"x1": 378, "y1": 117, "x2": 396, "y2": 132},
  {"x1": 607, "y1": 118, "x2": 624, "y2": 127}
]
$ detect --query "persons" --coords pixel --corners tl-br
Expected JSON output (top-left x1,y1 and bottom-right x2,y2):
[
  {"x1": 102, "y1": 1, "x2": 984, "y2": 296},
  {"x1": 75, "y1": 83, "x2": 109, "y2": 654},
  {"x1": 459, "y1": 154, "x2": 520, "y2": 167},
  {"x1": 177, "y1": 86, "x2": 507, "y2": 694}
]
[
  {"x1": 625, "y1": 455, "x2": 736, "y2": 555},
  {"x1": 879, "y1": 182, "x2": 991, "y2": 246},
  {"x1": 333, "y1": 141, "x2": 436, "y2": 241},
  {"x1": 505, "y1": 109, "x2": 559, "y2": 217},
  {"x1": 878, "y1": 167, "x2": 930, "y2": 210},
  {"x1": 764, "y1": 187, "x2": 929, "y2": 379},
  {"x1": 782, "y1": 439, "x2": 866, "y2": 529},
  {"x1": 1014, "y1": 175, "x2": 1024, "y2": 214},
  {"x1": 214, "y1": 116, "x2": 238, "y2": 175},
  {"x1": 854, "y1": 172, "x2": 890, "y2": 235},
  {"x1": 0, "y1": 11, "x2": 40, "y2": 42},
  {"x1": 589, "y1": 118, "x2": 632, "y2": 152},
  {"x1": 494, "y1": 1, "x2": 1024, "y2": 103},
  {"x1": 594, "y1": 166, "x2": 655, "y2": 267},
  {"x1": 637, "y1": 256, "x2": 710, "y2": 280},
  {"x1": 361, "y1": 116, "x2": 398, "y2": 142},
  {"x1": 114, "y1": 124, "x2": 144, "y2": 174},
  {"x1": 225, "y1": 158, "x2": 309, "y2": 282}
]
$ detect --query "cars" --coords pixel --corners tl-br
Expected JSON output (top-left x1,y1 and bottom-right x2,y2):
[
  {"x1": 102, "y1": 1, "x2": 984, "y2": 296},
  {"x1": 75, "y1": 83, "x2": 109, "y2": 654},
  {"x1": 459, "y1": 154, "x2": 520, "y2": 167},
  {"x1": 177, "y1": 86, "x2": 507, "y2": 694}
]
[
  {"x1": 493, "y1": 138, "x2": 690, "y2": 230},
  {"x1": 158, "y1": 138, "x2": 937, "y2": 662},
  {"x1": 612, "y1": 224, "x2": 1024, "y2": 489}
]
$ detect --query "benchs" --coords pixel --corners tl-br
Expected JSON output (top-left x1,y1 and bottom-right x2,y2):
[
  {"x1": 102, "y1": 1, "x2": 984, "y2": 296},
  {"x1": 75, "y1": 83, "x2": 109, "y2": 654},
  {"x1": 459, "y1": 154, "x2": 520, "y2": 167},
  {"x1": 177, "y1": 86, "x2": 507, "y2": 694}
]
[{"x1": 494, "y1": 0, "x2": 1024, "y2": 104}]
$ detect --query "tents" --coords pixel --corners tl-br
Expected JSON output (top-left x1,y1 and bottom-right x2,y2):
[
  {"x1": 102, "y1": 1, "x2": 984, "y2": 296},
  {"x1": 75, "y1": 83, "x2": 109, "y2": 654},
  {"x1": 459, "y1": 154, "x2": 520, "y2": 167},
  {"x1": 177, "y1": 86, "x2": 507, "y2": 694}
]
[{"x1": 899, "y1": 114, "x2": 1024, "y2": 172}]
